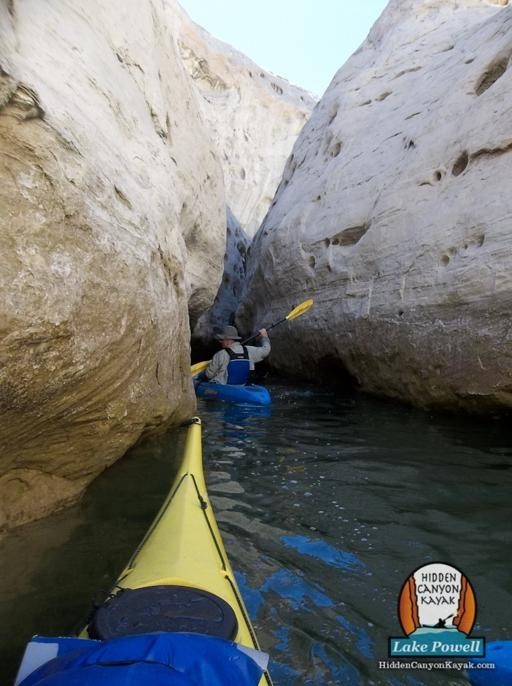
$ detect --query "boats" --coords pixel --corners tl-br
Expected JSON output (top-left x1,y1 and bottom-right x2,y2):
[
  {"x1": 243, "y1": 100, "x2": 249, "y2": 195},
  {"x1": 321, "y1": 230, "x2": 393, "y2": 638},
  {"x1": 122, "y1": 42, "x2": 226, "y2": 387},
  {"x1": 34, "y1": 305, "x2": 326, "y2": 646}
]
[
  {"x1": 66, "y1": 415, "x2": 272, "y2": 685},
  {"x1": 189, "y1": 372, "x2": 273, "y2": 405},
  {"x1": 468, "y1": 640, "x2": 512, "y2": 685}
]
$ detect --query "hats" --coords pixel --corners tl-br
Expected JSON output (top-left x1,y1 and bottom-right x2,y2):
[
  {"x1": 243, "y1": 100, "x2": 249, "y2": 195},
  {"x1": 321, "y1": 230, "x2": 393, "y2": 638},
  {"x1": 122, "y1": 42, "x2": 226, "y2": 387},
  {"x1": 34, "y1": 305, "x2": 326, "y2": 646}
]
[{"x1": 214, "y1": 326, "x2": 243, "y2": 340}]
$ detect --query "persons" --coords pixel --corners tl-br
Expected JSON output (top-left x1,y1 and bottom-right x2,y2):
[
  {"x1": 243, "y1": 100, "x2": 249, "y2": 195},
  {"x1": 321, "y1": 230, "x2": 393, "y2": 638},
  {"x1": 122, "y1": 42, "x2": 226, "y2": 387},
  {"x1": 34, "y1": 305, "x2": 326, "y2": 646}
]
[{"x1": 204, "y1": 325, "x2": 272, "y2": 386}]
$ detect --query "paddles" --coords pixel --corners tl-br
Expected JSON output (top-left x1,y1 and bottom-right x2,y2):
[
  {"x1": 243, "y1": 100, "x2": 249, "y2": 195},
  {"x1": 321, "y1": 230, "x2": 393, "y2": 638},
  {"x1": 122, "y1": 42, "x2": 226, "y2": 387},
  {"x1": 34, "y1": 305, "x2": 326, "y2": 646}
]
[{"x1": 191, "y1": 297, "x2": 316, "y2": 377}]
